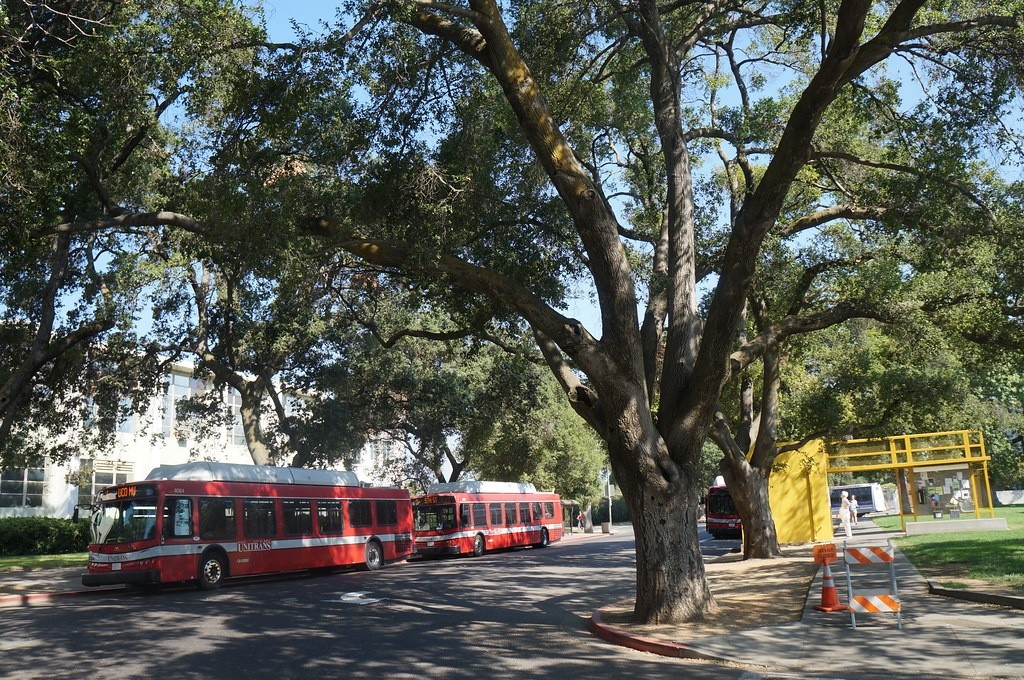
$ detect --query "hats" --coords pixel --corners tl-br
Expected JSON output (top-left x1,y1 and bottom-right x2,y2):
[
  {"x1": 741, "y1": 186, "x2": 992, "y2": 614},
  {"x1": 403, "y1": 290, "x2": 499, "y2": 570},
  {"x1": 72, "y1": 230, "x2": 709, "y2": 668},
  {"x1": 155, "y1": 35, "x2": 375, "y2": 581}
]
[{"x1": 841, "y1": 491, "x2": 848, "y2": 499}]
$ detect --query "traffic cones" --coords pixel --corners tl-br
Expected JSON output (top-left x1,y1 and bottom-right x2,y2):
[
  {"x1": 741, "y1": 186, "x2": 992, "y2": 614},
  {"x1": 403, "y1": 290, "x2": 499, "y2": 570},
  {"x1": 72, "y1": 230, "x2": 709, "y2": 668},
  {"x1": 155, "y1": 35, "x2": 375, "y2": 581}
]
[{"x1": 814, "y1": 558, "x2": 849, "y2": 613}]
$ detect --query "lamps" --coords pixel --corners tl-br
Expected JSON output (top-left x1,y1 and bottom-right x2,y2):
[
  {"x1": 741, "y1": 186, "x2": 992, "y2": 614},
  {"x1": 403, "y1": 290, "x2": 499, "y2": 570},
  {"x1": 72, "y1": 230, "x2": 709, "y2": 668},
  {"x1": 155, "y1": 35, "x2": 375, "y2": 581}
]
[{"x1": 933, "y1": 509, "x2": 943, "y2": 519}]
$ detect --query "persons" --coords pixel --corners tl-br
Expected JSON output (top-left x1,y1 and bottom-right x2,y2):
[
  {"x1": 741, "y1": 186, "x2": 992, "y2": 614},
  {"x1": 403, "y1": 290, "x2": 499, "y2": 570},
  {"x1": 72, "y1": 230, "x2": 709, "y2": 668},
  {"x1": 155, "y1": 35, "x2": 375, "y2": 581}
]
[
  {"x1": 840, "y1": 491, "x2": 852, "y2": 539},
  {"x1": 850, "y1": 495, "x2": 859, "y2": 523}
]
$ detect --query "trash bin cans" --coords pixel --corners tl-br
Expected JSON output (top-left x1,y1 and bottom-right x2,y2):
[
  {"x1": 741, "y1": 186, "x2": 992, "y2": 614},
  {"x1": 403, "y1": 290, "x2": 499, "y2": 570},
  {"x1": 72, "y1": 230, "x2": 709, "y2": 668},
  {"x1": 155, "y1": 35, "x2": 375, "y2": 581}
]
[
  {"x1": 933, "y1": 510, "x2": 943, "y2": 519},
  {"x1": 949, "y1": 509, "x2": 960, "y2": 519},
  {"x1": 601, "y1": 522, "x2": 610, "y2": 533}
]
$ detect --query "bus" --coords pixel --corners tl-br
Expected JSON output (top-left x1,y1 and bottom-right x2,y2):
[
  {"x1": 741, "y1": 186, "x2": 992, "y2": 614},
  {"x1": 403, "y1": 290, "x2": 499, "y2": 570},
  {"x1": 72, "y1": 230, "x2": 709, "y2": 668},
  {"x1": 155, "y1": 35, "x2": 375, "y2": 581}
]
[
  {"x1": 71, "y1": 459, "x2": 415, "y2": 591},
  {"x1": 405, "y1": 479, "x2": 565, "y2": 559},
  {"x1": 700, "y1": 474, "x2": 742, "y2": 540},
  {"x1": 829, "y1": 481, "x2": 886, "y2": 518}
]
